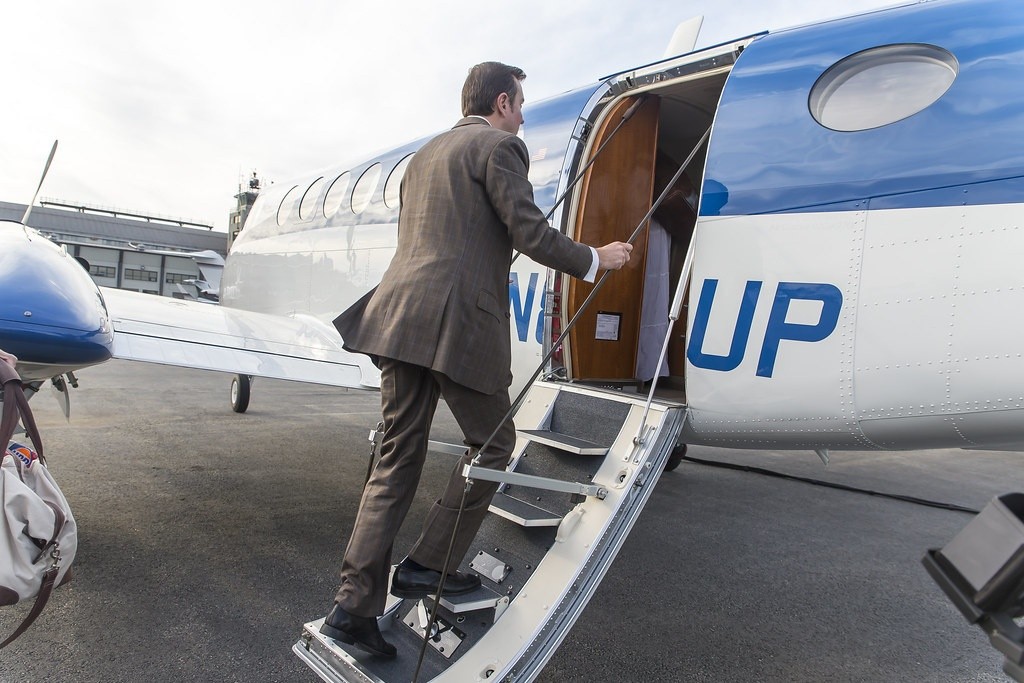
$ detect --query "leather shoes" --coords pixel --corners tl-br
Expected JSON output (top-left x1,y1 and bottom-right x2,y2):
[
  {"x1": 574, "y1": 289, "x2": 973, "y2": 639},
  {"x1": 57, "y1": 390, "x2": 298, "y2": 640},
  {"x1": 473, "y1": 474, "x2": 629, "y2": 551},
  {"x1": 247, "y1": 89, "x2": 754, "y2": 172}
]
[
  {"x1": 390, "y1": 564, "x2": 481, "y2": 599},
  {"x1": 320, "y1": 602, "x2": 396, "y2": 659}
]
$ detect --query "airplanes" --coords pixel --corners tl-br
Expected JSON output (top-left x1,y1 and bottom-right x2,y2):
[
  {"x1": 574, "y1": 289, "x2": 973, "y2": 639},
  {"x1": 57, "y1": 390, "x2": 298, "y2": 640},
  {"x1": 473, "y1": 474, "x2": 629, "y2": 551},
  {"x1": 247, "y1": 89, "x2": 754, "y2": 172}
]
[{"x1": 0, "y1": 1, "x2": 1021, "y2": 683}]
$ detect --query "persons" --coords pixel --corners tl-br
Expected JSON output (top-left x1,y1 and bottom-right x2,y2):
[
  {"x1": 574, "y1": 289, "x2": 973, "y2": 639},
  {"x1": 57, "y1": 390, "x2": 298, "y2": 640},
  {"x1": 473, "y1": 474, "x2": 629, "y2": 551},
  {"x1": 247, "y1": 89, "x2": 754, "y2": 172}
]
[{"x1": 320, "y1": 62, "x2": 633, "y2": 658}]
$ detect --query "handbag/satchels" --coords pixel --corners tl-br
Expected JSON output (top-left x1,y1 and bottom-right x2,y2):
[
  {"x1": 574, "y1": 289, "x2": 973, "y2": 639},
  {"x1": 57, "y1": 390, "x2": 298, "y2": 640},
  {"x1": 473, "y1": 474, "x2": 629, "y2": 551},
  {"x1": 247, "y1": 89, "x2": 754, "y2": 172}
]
[{"x1": 0, "y1": 351, "x2": 78, "y2": 650}]
